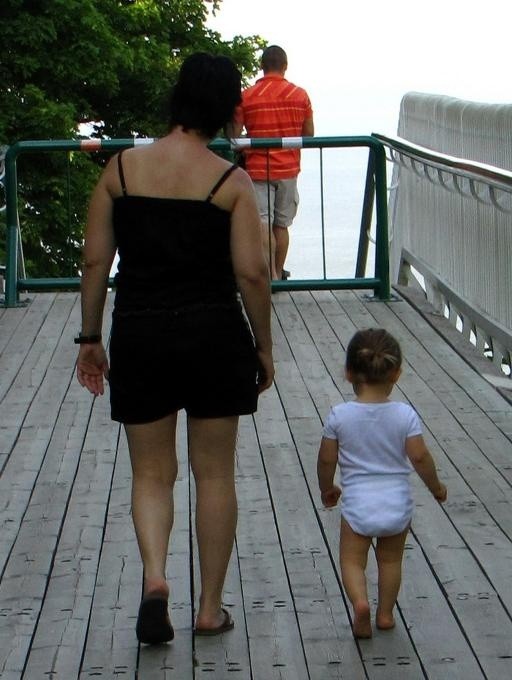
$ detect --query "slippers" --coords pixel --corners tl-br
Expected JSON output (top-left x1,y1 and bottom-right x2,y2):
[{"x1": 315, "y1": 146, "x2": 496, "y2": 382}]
[
  {"x1": 137, "y1": 597, "x2": 174, "y2": 643},
  {"x1": 194, "y1": 608, "x2": 235, "y2": 635}
]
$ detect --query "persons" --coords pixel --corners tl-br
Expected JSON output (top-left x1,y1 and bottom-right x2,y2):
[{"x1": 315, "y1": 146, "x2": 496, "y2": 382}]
[
  {"x1": 73, "y1": 46, "x2": 279, "y2": 645},
  {"x1": 226, "y1": 40, "x2": 316, "y2": 284},
  {"x1": 314, "y1": 326, "x2": 450, "y2": 642}
]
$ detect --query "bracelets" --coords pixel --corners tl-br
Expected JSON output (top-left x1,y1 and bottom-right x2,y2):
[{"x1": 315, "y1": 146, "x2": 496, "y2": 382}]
[{"x1": 74, "y1": 333, "x2": 104, "y2": 346}]
